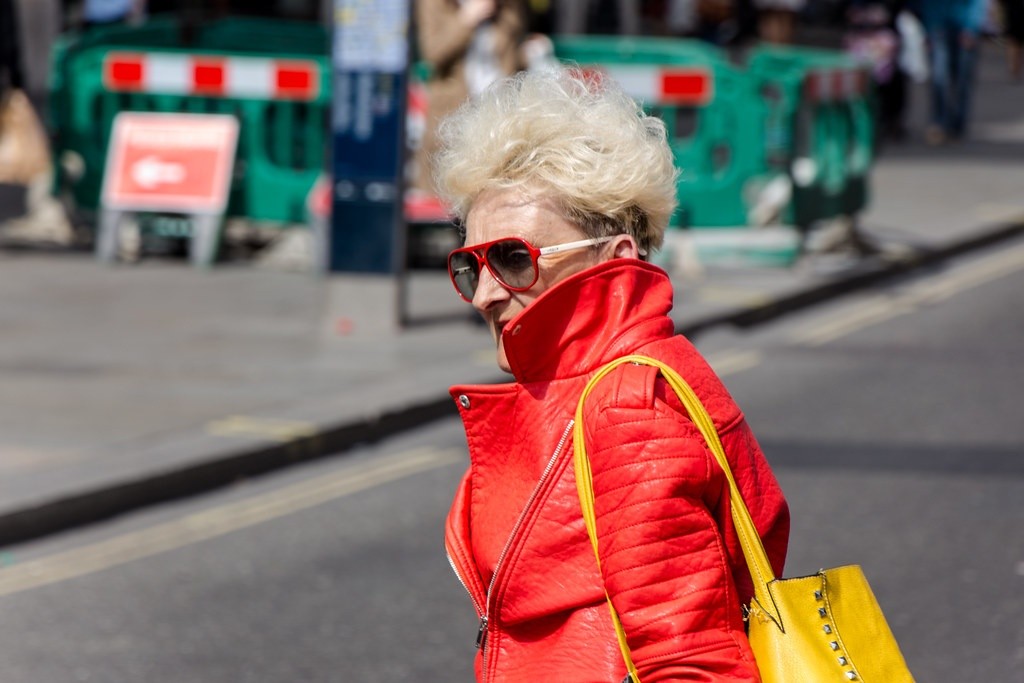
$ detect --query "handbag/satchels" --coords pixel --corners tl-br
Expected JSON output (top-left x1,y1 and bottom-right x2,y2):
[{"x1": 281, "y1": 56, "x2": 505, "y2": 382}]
[
  {"x1": 572, "y1": 353, "x2": 916, "y2": 683},
  {"x1": 983, "y1": 0, "x2": 1005, "y2": 36}
]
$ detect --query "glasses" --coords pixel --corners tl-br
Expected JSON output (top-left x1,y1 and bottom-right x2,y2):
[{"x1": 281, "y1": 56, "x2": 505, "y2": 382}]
[{"x1": 447, "y1": 237, "x2": 648, "y2": 304}]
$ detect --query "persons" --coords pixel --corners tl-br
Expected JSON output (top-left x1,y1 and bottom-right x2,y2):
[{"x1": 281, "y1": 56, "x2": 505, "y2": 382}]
[
  {"x1": 431, "y1": 62, "x2": 791, "y2": 683},
  {"x1": 413, "y1": 1, "x2": 531, "y2": 204},
  {"x1": 669, "y1": 0, "x2": 990, "y2": 146}
]
